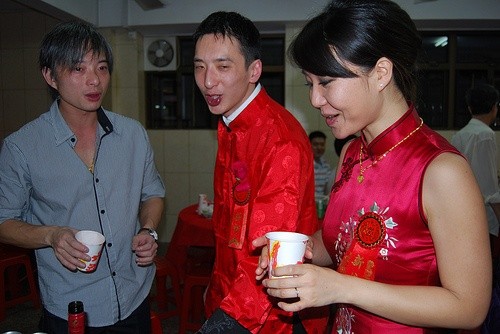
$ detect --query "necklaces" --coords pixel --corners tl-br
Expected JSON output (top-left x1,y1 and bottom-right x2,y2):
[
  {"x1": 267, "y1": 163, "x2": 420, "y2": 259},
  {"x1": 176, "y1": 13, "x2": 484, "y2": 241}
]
[
  {"x1": 88, "y1": 162, "x2": 95, "y2": 174},
  {"x1": 359, "y1": 116, "x2": 424, "y2": 183}
]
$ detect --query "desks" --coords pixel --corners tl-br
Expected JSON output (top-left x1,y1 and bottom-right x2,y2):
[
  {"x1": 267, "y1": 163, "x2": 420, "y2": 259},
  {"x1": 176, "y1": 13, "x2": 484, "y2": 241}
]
[{"x1": 168, "y1": 203, "x2": 215, "y2": 321}]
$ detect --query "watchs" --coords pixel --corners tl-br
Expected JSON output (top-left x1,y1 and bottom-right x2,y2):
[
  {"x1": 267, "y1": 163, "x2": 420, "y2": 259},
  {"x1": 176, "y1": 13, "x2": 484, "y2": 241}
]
[{"x1": 136, "y1": 227, "x2": 158, "y2": 241}]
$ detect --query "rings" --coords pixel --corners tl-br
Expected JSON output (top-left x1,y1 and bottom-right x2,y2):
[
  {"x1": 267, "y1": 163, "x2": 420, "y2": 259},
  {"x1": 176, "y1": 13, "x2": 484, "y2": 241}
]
[{"x1": 295, "y1": 287, "x2": 299, "y2": 298}]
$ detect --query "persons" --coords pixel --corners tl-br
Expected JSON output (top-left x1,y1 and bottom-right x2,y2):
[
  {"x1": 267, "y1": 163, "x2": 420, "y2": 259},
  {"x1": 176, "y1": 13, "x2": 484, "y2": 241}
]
[
  {"x1": 0, "y1": 20, "x2": 164, "y2": 334},
  {"x1": 194, "y1": 11, "x2": 328, "y2": 334},
  {"x1": 310, "y1": 132, "x2": 358, "y2": 220},
  {"x1": 450, "y1": 81, "x2": 500, "y2": 282},
  {"x1": 252, "y1": 1, "x2": 493, "y2": 334}
]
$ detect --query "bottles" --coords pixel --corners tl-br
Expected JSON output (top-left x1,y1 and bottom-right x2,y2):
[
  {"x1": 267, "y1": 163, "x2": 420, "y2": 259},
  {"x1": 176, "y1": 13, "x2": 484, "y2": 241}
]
[{"x1": 68, "y1": 301, "x2": 85, "y2": 334}]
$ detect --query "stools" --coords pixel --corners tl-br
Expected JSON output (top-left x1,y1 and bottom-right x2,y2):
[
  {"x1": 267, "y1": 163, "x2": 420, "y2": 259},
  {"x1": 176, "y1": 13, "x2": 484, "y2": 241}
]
[
  {"x1": 0, "y1": 246, "x2": 40, "y2": 321},
  {"x1": 149, "y1": 242, "x2": 211, "y2": 334}
]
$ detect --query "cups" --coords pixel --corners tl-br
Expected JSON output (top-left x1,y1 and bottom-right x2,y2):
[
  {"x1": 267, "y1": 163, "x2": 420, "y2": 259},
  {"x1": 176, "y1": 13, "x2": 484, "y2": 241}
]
[
  {"x1": 74, "y1": 231, "x2": 105, "y2": 272},
  {"x1": 198, "y1": 194, "x2": 208, "y2": 212},
  {"x1": 265, "y1": 231, "x2": 310, "y2": 280}
]
